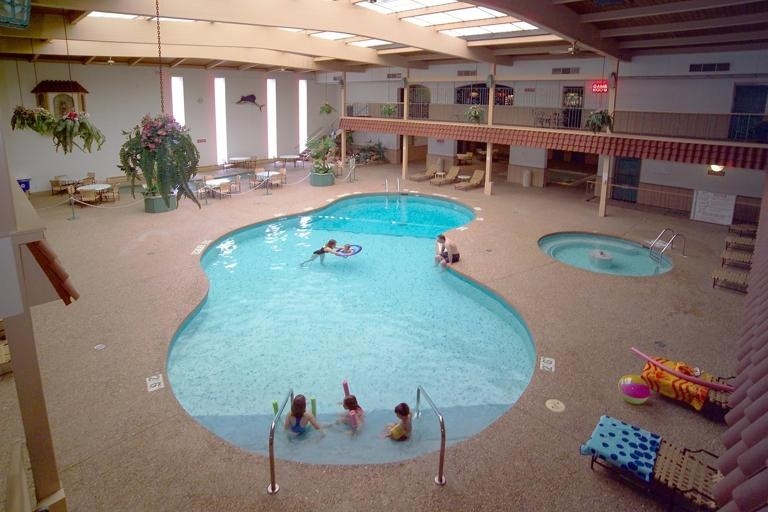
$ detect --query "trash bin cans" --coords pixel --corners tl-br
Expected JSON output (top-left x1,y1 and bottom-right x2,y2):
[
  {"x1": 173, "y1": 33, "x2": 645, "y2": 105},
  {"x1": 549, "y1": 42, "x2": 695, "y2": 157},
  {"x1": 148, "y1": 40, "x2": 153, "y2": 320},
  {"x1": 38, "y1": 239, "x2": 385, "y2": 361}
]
[
  {"x1": 436, "y1": 158, "x2": 443, "y2": 173},
  {"x1": 522, "y1": 169, "x2": 531, "y2": 187},
  {"x1": 16, "y1": 178, "x2": 30, "y2": 199}
]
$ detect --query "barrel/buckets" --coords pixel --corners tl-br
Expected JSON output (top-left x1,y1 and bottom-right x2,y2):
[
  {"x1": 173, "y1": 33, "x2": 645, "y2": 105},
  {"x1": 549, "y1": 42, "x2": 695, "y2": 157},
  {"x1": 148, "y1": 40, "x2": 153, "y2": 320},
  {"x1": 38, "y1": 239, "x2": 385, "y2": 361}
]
[{"x1": 16, "y1": 176, "x2": 32, "y2": 202}]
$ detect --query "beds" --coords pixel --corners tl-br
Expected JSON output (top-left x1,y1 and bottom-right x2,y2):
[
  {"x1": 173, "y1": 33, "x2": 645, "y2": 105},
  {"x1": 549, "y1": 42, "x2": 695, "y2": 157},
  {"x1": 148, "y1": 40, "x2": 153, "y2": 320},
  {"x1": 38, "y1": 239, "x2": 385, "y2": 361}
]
[
  {"x1": 583, "y1": 354, "x2": 736, "y2": 508},
  {"x1": 713, "y1": 223, "x2": 759, "y2": 293}
]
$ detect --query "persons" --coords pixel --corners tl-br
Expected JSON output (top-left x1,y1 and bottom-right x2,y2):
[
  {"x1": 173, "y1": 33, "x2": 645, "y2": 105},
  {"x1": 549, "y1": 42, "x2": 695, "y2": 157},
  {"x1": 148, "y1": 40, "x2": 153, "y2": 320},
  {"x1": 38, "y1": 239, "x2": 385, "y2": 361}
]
[
  {"x1": 335, "y1": 394, "x2": 365, "y2": 436},
  {"x1": 434, "y1": 235, "x2": 460, "y2": 271},
  {"x1": 340, "y1": 244, "x2": 353, "y2": 254},
  {"x1": 283, "y1": 394, "x2": 326, "y2": 440},
  {"x1": 300, "y1": 239, "x2": 340, "y2": 267},
  {"x1": 376, "y1": 403, "x2": 412, "y2": 441}
]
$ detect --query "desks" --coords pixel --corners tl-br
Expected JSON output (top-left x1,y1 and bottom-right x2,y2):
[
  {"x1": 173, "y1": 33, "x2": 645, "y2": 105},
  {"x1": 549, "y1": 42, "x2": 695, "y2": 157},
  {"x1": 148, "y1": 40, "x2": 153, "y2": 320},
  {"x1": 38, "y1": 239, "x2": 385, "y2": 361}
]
[
  {"x1": 279, "y1": 155, "x2": 300, "y2": 168},
  {"x1": 229, "y1": 157, "x2": 250, "y2": 169}
]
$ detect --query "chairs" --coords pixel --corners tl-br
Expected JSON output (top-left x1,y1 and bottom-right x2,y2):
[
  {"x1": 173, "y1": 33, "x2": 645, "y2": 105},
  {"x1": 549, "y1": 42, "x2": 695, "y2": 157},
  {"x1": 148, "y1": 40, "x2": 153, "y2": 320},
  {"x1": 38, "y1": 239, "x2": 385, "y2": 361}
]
[
  {"x1": 49, "y1": 172, "x2": 120, "y2": 207},
  {"x1": 195, "y1": 167, "x2": 287, "y2": 200},
  {"x1": 456, "y1": 151, "x2": 473, "y2": 165},
  {"x1": 409, "y1": 164, "x2": 486, "y2": 190}
]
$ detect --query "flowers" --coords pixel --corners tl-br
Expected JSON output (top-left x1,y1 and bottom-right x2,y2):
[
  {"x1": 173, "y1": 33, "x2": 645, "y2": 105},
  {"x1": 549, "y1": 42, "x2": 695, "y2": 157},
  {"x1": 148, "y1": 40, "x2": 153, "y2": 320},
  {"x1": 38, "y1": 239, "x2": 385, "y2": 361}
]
[
  {"x1": 118, "y1": 110, "x2": 203, "y2": 210},
  {"x1": 11, "y1": 104, "x2": 106, "y2": 155}
]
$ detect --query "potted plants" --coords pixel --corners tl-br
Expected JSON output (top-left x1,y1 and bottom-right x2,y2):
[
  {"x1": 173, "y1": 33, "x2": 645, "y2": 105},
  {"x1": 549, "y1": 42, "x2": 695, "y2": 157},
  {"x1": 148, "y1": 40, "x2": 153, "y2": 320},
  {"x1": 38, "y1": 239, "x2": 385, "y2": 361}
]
[
  {"x1": 305, "y1": 138, "x2": 337, "y2": 186},
  {"x1": 585, "y1": 108, "x2": 616, "y2": 135}
]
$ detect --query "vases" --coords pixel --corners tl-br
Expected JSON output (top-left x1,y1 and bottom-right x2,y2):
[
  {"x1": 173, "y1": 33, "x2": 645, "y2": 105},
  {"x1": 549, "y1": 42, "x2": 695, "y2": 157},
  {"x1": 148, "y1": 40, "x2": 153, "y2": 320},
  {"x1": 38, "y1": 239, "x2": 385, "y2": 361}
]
[{"x1": 145, "y1": 191, "x2": 179, "y2": 213}]
